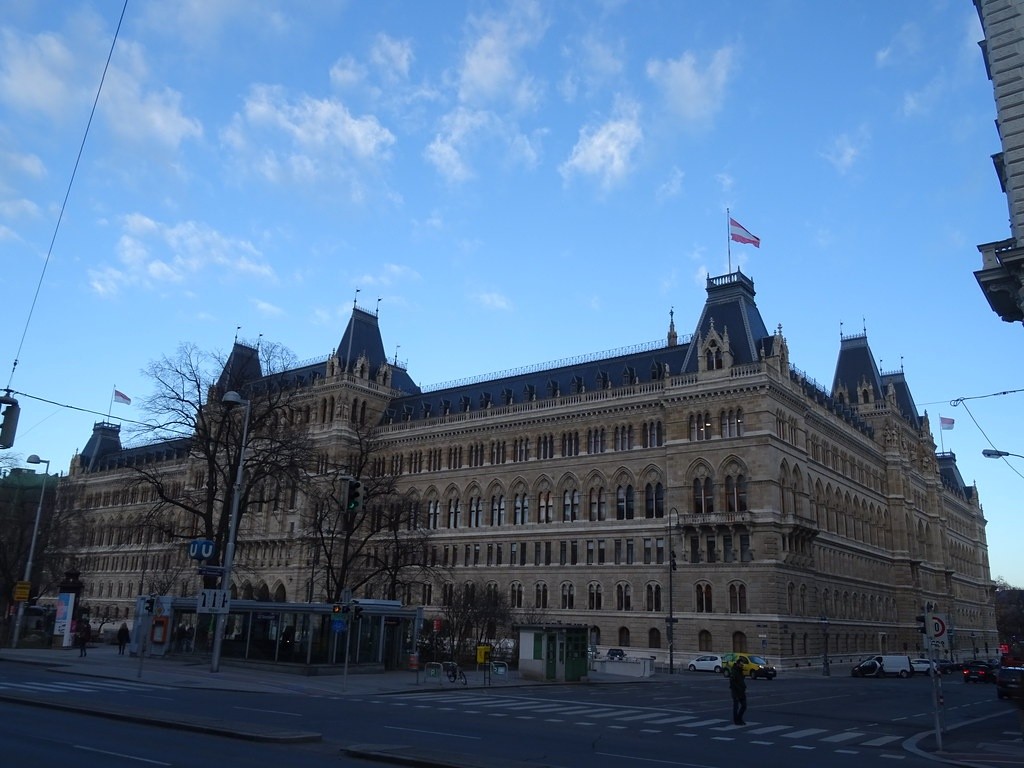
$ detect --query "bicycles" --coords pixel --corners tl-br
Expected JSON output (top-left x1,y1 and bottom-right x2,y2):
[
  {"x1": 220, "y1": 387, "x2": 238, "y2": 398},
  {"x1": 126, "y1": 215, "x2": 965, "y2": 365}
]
[{"x1": 446, "y1": 662, "x2": 468, "y2": 685}]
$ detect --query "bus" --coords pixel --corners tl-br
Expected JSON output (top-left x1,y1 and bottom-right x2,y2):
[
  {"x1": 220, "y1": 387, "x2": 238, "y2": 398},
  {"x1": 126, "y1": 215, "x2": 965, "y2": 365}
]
[{"x1": 24, "y1": 605, "x2": 50, "y2": 631}]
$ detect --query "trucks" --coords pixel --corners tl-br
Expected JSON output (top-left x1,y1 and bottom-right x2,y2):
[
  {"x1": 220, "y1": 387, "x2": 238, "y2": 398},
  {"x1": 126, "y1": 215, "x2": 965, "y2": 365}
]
[{"x1": 852, "y1": 654, "x2": 916, "y2": 679}]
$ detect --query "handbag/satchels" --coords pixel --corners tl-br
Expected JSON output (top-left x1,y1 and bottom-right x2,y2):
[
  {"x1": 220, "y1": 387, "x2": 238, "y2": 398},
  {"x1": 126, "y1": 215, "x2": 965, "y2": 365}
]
[{"x1": 126, "y1": 638, "x2": 131, "y2": 643}]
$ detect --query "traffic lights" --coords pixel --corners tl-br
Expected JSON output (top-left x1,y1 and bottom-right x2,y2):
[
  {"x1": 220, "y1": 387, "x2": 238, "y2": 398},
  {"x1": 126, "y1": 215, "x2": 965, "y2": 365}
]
[
  {"x1": 354, "y1": 606, "x2": 363, "y2": 624},
  {"x1": 346, "y1": 481, "x2": 363, "y2": 511},
  {"x1": 915, "y1": 615, "x2": 928, "y2": 634},
  {"x1": 333, "y1": 605, "x2": 341, "y2": 613}
]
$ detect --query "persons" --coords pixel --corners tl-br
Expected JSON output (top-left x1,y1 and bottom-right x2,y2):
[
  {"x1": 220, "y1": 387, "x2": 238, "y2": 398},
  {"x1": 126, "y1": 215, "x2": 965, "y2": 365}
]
[
  {"x1": 117, "y1": 623, "x2": 130, "y2": 655},
  {"x1": 729, "y1": 659, "x2": 747, "y2": 726},
  {"x1": 79, "y1": 620, "x2": 91, "y2": 657}
]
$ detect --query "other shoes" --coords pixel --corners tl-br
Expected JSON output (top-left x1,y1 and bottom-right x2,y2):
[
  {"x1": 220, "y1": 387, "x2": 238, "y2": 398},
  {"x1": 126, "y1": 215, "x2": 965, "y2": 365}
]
[{"x1": 734, "y1": 719, "x2": 746, "y2": 725}]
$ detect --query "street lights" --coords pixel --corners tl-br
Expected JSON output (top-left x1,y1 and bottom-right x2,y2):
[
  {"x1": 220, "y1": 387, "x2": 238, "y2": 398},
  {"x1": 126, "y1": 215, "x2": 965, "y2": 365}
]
[
  {"x1": 11, "y1": 455, "x2": 54, "y2": 645},
  {"x1": 818, "y1": 617, "x2": 831, "y2": 676},
  {"x1": 209, "y1": 391, "x2": 254, "y2": 672},
  {"x1": 665, "y1": 506, "x2": 682, "y2": 673}
]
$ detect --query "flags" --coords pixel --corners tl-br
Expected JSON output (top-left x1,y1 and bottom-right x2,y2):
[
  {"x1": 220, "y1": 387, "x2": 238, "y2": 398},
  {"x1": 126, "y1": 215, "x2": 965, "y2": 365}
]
[
  {"x1": 115, "y1": 390, "x2": 131, "y2": 405},
  {"x1": 730, "y1": 218, "x2": 759, "y2": 248},
  {"x1": 941, "y1": 418, "x2": 954, "y2": 430}
]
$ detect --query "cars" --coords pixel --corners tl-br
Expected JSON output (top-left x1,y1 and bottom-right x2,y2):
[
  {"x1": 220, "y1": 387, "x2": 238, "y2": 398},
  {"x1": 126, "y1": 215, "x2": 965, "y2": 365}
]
[
  {"x1": 961, "y1": 659, "x2": 999, "y2": 684},
  {"x1": 688, "y1": 655, "x2": 723, "y2": 672},
  {"x1": 606, "y1": 648, "x2": 625, "y2": 660}
]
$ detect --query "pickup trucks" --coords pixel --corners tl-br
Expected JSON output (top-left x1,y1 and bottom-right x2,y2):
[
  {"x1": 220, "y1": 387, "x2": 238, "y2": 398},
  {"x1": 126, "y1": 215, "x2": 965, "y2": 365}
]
[{"x1": 722, "y1": 653, "x2": 778, "y2": 680}]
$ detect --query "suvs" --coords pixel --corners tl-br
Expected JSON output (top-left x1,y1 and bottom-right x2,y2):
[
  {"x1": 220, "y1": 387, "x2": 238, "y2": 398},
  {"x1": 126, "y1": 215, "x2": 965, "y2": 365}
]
[
  {"x1": 997, "y1": 666, "x2": 1024, "y2": 702},
  {"x1": 911, "y1": 658, "x2": 937, "y2": 675},
  {"x1": 934, "y1": 659, "x2": 953, "y2": 674}
]
